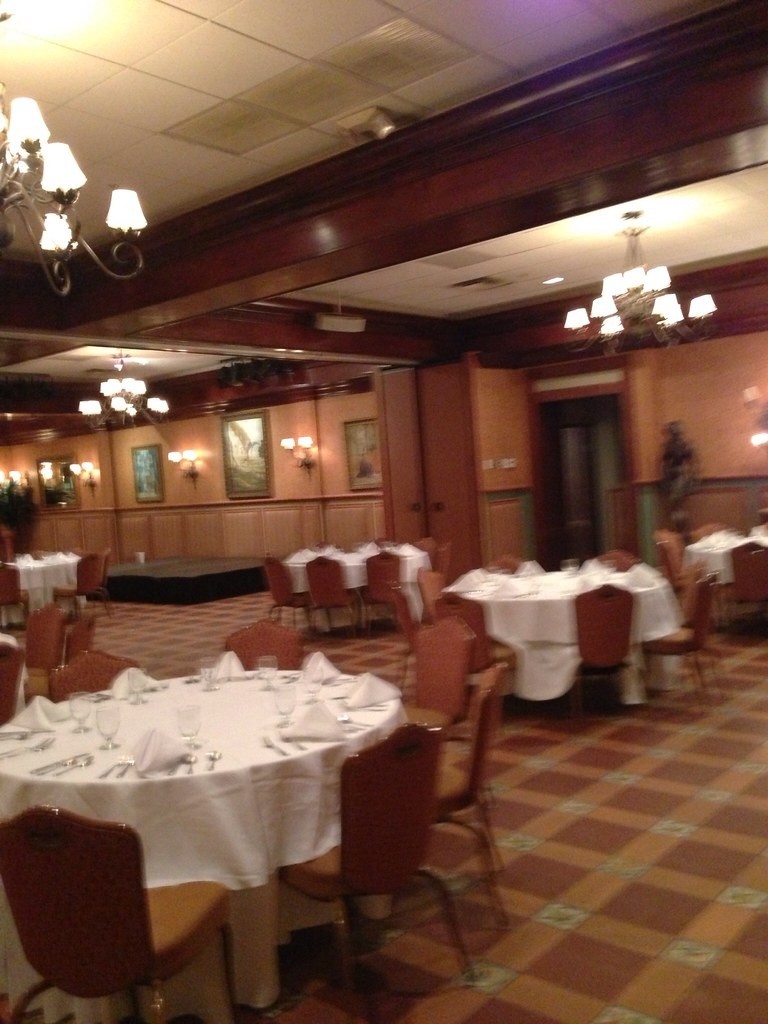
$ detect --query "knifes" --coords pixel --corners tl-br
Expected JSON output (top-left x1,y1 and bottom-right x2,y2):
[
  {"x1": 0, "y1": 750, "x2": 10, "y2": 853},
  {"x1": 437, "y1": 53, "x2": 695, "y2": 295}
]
[{"x1": 30, "y1": 753, "x2": 90, "y2": 774}]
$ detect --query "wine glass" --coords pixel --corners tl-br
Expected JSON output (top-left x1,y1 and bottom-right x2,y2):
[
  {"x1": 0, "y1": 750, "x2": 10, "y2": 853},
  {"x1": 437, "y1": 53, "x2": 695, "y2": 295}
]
[
  {"x1": 274, "y1": 685, "x2": 297, "y2": 728},
  {"x1": 68, "y1": 692, "x2": 92, "y2": 733},
  {"x1": 127, "y1": 668, "x2": 150, "y2": 706},
  {"x1": 199, "y1": 656, "x2": 219, "y2": 692},
  {"x1": 95, "y1": 705, "x2": 122, "y2": 752},
  {"x1": 256, "y1": 656, "x2": 278, "y2": 691},
  {"x1": 177, "y1": 704, "x2": 203, "y2": 755},
  {"x1": 302, "y1": 667, "x2": 324, "y2": 706}
]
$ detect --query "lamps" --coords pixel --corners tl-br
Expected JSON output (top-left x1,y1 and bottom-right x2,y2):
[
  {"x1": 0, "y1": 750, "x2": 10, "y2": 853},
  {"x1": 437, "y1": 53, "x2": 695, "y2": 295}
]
[
  {"x1": 168, "y1": 450, "x2": 198, "y2": 478},
  {"x1": 216, "y1": 354, "x2": 296, "y2": 387},
  {"x1": 70, "y1": 461, "x2": 98, "y2": 488},
  {"x1": 0, "y1": 82, "x2": 148, "y2": 296},
  {"x1": 280, "y1": 436, "x2": 313, "y2": 468},
  {"x1": 0, "y1": 471, "x2": 21, "y2": 497},
  {"x1": 564, "y1": 209, "x2": 718, "y2": 352},
  {"x1": 78, "y1": 356, "x2": 170, "y2": 429}
]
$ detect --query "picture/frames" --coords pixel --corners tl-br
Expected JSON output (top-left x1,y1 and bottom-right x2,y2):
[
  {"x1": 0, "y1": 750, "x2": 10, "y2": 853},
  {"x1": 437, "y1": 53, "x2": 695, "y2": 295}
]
[
  {"x1": 132, "y1": 444, "x2": 163, "y2": 502},
  {"x1": 221, "y1": 409, "x2": 270, "y2": 498},
  {"x1": 343, "y1": 417, "x2": 382, "y2": 490}
]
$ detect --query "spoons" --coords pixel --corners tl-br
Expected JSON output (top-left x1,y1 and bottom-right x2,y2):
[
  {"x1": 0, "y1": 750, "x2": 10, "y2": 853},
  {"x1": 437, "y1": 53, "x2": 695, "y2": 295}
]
[
  {"x1": 184, "y1": 756, "x2": 197, "y2": 775},
  {"x1": 209, "y1": 751, "x2": 221, "y2": 771},
  {"x1": 37, "y1": 756, "x2": 95, "y2": 776}
]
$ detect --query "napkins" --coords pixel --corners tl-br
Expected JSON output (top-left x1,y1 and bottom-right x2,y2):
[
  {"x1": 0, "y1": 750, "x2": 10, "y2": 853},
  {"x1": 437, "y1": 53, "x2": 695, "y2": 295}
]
[
  {"x1": 345, "y1": 671, "x2": 403, "y2": 707},
  {"x1": 212, "y1": 652, "x2": 248, "y2": 681},
  {"x1": 12, "y1": 694, "x2": 72, "y2": 731},
  {"x1": 281, "y1": 699, "x2": 346, "y2": 743},
  {"x1": 113, "y1": 667, "x2": 163, "y2": 697},
  {"x1": 300, "y1": 652, "x2": 342, "y2": 685},
  {"x1": 136, "y1": 720, "x2": 187, "y2": 778}
]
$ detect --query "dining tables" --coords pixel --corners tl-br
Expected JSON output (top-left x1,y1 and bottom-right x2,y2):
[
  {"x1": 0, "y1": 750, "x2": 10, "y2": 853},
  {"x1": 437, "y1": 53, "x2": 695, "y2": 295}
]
[
  {"x1": 684, "y1": 526, "x2": 768, "y2": 625},
  {"x1": 443, "y1": 563, "x2": 690, "y2": 703},
  {"x1": 284, "y1": 545, "x2": 431, "y2": 635},
  {"x1": 0, "y1": 666, "x2": 411, "y2": 1005},
  {"x1": 7, "y1": 550, "x2": 81, "y2": 615}
]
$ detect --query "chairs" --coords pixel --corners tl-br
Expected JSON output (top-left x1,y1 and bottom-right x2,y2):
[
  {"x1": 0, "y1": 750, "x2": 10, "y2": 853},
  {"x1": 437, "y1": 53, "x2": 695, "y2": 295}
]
[{"x1": 0, "y1": 518, "x2": 768, "y2": 1024}]
